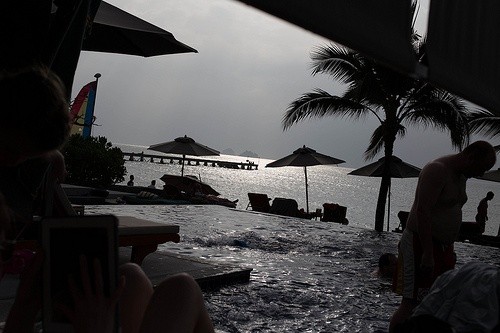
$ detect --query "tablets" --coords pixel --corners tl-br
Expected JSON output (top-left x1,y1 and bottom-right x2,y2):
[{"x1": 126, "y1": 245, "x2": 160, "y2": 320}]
[{"x1": 40, "y1": 215, "x2": 119, "y2": 333}]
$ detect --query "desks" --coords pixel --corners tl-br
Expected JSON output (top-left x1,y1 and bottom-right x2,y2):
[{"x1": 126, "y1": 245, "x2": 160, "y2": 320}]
[{"x1": 8, "y1": 217, "x2": 180, "y2": 270}]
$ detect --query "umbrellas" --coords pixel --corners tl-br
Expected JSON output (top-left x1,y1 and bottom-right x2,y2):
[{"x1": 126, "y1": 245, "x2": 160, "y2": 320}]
[
  {"x1": 348, "y1": 155, "x2": 423, "y2": 232},
  {"x1": 266, "y1": 145, "x2": 346, "y2": 214},
  {"x1": 148, "y1": 135, "x2": 220, "y2": 177},
  {"x1": 81, "y1": 1, "x2": 197, "y2": 57}
]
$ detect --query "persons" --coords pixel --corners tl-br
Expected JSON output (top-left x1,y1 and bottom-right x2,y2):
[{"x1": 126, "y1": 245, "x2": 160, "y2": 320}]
[
  {"x1": 0, "y1": 64, "x2": 215, "y2": 333},
  {"x1": 388, "y1": 141, "x2": 500, "y2": 333}
]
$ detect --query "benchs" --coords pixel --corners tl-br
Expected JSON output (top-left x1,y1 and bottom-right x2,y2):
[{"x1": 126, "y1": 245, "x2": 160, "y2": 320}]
[
  {"x1": 270, "y1": 198, "x2": 307, "y2": 218},
  {"x1": 321, "y1": 203, "x2": 349, "y2": 225}
]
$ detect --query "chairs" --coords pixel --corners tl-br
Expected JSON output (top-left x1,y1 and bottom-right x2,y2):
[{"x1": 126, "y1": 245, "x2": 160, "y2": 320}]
[
  {"x1": 245, "y1": 192, "x2": 272, "y2": 213},
  {"x1": 391, "y1": 211, "x2": 410, "y2": 233}
]
